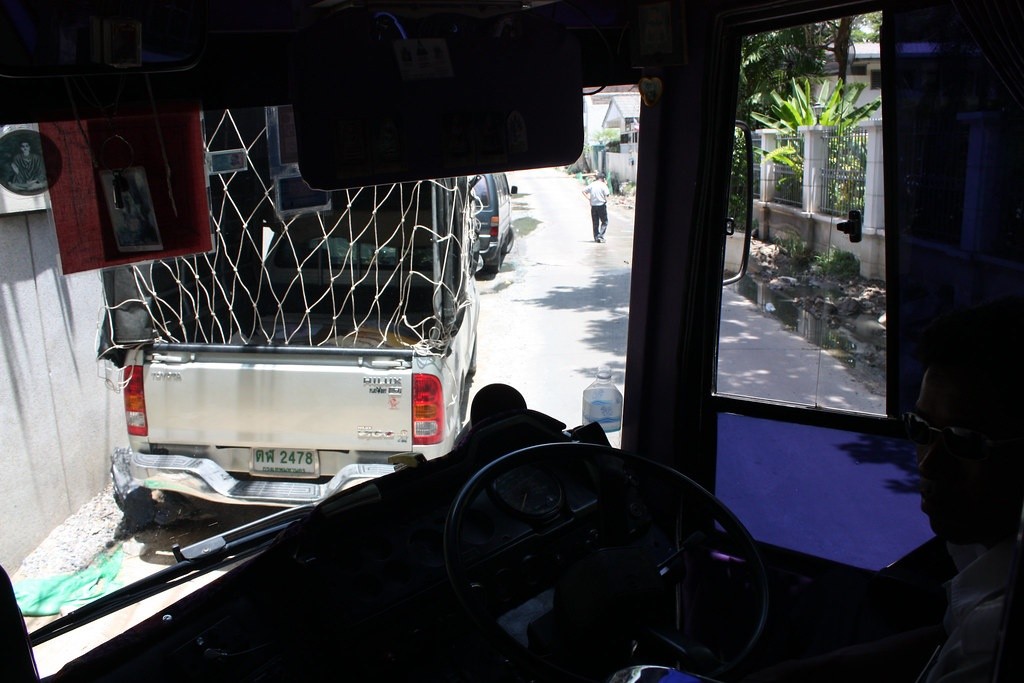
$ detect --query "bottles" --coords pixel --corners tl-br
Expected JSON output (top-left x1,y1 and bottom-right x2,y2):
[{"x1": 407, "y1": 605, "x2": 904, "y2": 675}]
[{"x1": 582, "y1": 366, "x2": 623, "y2": 449}]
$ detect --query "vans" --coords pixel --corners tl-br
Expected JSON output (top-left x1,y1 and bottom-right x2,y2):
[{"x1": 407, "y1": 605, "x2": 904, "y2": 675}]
[{"x1": 465, "y1": 173, "x2": 519, "y2": 274}]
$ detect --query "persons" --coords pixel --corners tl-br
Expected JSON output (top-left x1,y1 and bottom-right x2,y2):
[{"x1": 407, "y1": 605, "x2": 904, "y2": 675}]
[
  {"x1": 10, "y1": 140, "x2": 43, "y2": 187},
  {"x1": 583, "y1": 173, "x2": 610, "y2": 242},
  {"x1": 747, "y1": 293, "x2": 1024, "y2": 683}
]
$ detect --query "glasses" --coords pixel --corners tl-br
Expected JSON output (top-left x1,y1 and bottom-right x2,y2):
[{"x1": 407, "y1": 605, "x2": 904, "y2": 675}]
[{"x1": 900, "y1": 409, "x2": 1021, "y2": 462}]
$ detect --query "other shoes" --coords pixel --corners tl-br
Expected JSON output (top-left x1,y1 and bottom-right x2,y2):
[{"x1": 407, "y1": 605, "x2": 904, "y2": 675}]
[{"x1": 596, "y1": 235, "x2": 607, "y2": 244}]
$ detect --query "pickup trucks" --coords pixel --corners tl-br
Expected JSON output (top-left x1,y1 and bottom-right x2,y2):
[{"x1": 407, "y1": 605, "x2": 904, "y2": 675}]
[{"x1": 100, "y1": 97, "x2": 485, "y2": 530}]
